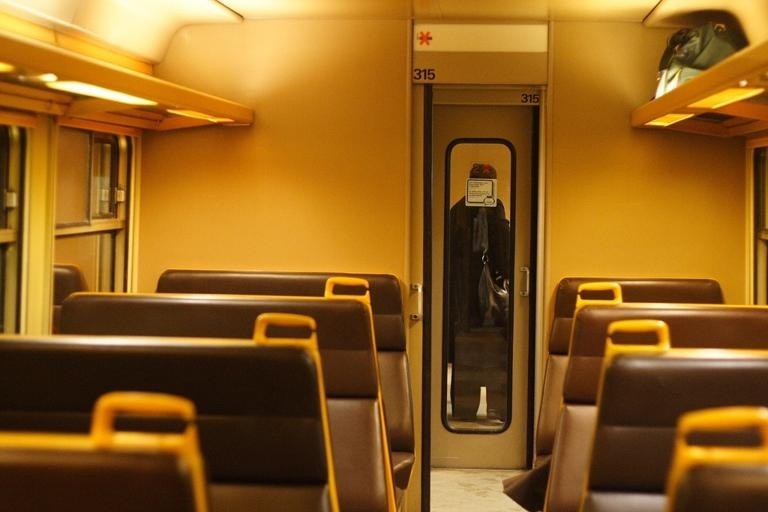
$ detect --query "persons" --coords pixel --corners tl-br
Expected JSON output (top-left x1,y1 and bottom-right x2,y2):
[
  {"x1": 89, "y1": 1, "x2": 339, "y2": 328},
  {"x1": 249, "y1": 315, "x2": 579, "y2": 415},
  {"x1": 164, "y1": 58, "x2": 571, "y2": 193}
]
[{"x1": 447, "y1": 164, "x2": 510, "y2": 425}]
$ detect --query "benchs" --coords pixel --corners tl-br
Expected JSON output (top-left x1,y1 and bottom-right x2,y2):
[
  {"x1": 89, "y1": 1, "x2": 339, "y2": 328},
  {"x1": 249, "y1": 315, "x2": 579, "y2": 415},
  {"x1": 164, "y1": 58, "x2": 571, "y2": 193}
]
[
  {"x1": 0, "y1": 265, "x2": 415, "y2": 512},
  {"x1": 519, "y1": 275, "x2": 768, "y2": 511}
]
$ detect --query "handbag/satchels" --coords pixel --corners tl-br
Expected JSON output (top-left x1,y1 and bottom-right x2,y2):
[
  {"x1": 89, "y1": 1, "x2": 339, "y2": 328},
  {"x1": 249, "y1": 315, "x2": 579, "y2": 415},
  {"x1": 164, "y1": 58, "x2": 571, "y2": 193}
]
[
  {"x1": 655, "y1": 23, "x2": 737, "y2": 120},
  {"x1": 479, "y1": 254, "x2": 509, "y2": 327}
]
